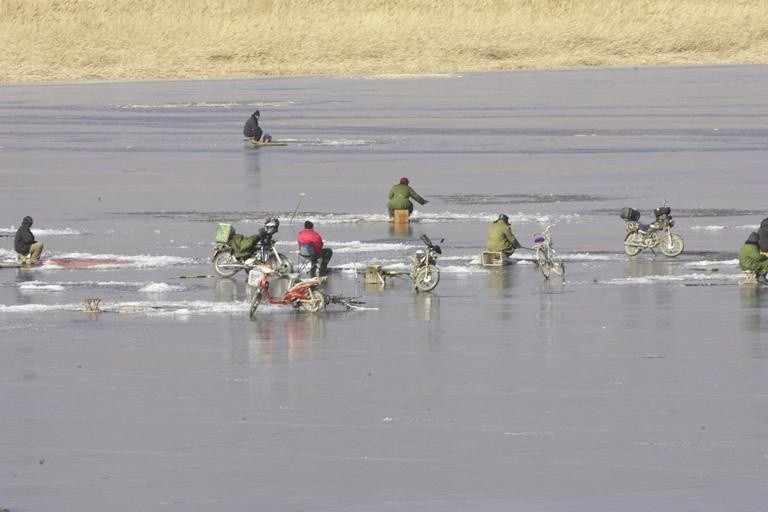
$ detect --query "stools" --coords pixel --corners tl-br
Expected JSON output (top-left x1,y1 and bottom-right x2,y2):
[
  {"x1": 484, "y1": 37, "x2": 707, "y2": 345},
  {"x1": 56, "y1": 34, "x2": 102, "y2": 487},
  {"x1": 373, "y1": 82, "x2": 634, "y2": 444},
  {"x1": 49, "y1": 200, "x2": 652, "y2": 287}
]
[
  {"x1": 394, "y1": 209, "x2": 410, "y2": 223},
  {"x1": 479, "y1": 251, "x2": 510, "y2": 267},
  {"x1": 297, "y1": 254, "x2": 318, "y2": 273},
  {"x1": 740, "y1": 269, "x2": 759, "y2": 285},
  {"x1": 84, "y1": 298, "x2": 103, "y2": 314},
  {"x1": 15, "y1": 252, "x2": 28, "y2": 265}
]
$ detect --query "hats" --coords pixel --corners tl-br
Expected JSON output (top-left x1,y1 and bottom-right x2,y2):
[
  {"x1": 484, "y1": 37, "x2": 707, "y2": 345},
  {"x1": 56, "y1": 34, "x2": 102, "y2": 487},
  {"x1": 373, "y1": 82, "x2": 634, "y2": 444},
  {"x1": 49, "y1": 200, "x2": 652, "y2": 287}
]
[
  {"x1": 400, "y1": 177, "x2": 409, "y2": 184},
  {"x1": 748, "y1": 232, "x2": 759, "y2": 243},
  {"x1": 500, "y1": 214, "x2": 508, "y2": 221},
  {"x1": 254, "y1": 111, "x2": 260, "y2": 116}
]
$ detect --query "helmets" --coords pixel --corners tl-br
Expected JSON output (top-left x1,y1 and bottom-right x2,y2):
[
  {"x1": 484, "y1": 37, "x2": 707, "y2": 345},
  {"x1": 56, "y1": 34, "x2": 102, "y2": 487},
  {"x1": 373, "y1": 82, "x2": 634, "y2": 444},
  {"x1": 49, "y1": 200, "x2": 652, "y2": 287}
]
[{"x1": 265, "y1": 219, "x2": 279, "y2": 227}]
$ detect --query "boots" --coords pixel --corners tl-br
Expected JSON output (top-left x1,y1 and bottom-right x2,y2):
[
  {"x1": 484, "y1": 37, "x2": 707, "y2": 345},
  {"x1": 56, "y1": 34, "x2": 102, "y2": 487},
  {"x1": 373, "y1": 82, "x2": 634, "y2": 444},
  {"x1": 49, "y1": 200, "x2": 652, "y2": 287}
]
[{"x1": 759, "y1": 275, "x2": 768, "y2": 284}]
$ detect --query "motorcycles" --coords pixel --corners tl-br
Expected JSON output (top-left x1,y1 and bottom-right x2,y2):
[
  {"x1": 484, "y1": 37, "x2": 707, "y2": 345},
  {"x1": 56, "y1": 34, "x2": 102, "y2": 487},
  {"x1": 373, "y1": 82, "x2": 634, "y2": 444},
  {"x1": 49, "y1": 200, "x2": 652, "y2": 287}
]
[
  {"x1": 210, "y1": 217, "x2": 293, "y2": 279},
  {"x1": 619, "y1": 199, "x2": 684, "y2": 257},
  {"x1": 247, "y1": 264, "x2": 329, "y2": 316},
  {"x1": 410, "y1": 233, "x2": 446, "y2": 291}
]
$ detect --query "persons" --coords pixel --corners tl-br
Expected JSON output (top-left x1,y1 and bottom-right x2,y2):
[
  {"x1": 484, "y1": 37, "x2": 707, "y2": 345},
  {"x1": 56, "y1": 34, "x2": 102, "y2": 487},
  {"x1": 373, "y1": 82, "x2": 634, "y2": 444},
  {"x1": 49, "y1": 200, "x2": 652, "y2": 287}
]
[
  {"x1": 14, "y1": 215, "x2": 44, "y2": 266},
  {"x1": 297, "y1": 221, "x2": 333, "y2": 273},
  {"x1": 486, "y1": 214, "x2": 521, "y2": 264},
  {"x1": 243, "y1": 110, "x2": 263, "y2": 142},
  {"x1": 739, "y1": 218, "x2": 768, "y2": 286},
  {"x1": 388, "y1": 177, "x2": 429, "y2": 219}
]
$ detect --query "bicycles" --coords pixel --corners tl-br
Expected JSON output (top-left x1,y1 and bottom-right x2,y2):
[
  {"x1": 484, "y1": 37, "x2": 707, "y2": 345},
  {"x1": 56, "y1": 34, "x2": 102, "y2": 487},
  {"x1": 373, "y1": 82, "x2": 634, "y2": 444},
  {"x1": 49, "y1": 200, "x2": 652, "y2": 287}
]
[
  {"x1": 322, "y1": 291, "x2": 366, "y2": 310},
  {"x1": 531, "y1": 223, "x2": 566, "y2": 277},
  {"x1": 358, "y1": 265, "x2": 411, "y2": 287}
]
